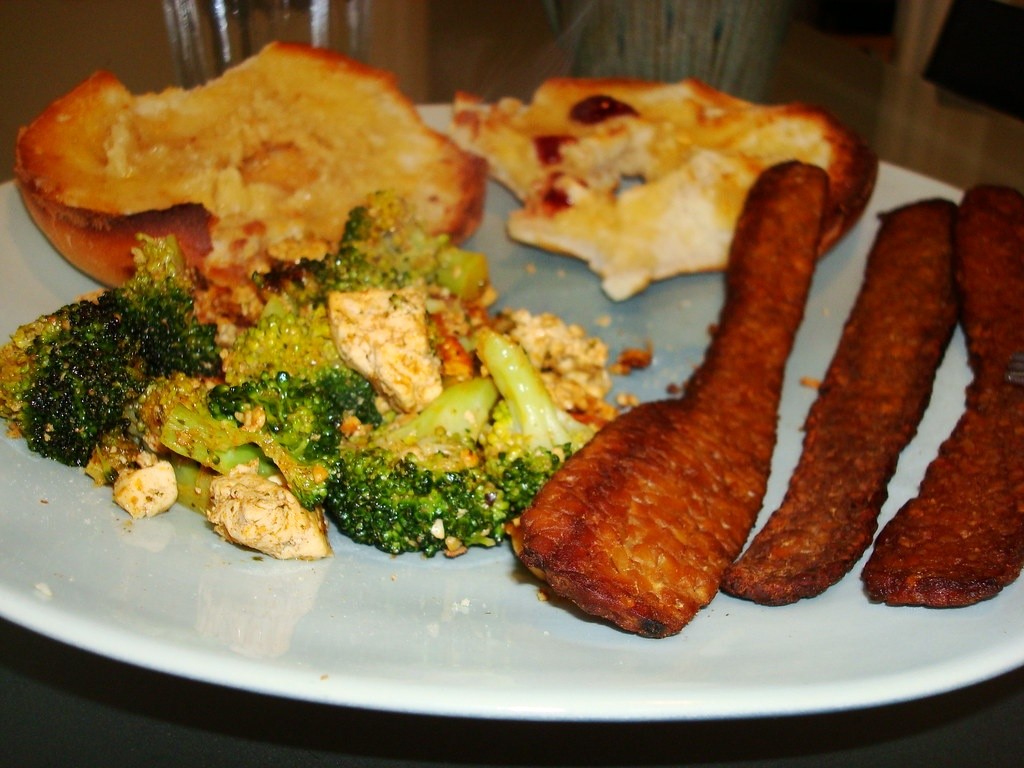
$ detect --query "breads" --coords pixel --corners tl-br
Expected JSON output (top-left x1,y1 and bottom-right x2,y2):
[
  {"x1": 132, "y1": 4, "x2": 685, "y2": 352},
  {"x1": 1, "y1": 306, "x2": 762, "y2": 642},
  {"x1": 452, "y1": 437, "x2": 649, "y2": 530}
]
[
  {"x1": 8, "y1": 42, "x2": 489, "y2": 313},
  {"x1": 450, "y1": 71, "x2": 878, "y2": 300}
]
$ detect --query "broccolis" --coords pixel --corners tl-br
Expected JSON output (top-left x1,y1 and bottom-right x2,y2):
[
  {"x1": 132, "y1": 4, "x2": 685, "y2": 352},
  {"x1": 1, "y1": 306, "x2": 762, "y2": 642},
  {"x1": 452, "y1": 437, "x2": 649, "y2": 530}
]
[{"x1": 0, "y1": 189, "x2": 599, "y2": 562}]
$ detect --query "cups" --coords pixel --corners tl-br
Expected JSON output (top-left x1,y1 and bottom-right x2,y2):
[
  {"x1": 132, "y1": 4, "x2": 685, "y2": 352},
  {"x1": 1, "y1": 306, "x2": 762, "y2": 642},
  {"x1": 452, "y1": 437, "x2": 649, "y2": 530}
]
[
  {"x1": 163, "y1": 0, "x2": 432, "y2": 104},
  {"x1": 542, "y1": 0, "x2": 792, "y2": 101}
]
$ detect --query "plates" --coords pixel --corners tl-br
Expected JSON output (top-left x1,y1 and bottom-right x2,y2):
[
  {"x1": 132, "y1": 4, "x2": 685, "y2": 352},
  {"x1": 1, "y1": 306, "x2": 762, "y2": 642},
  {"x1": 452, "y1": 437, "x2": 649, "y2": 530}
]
[{"x1": 0, "y1": 98, "x2": 1024, "y2": 721}]
